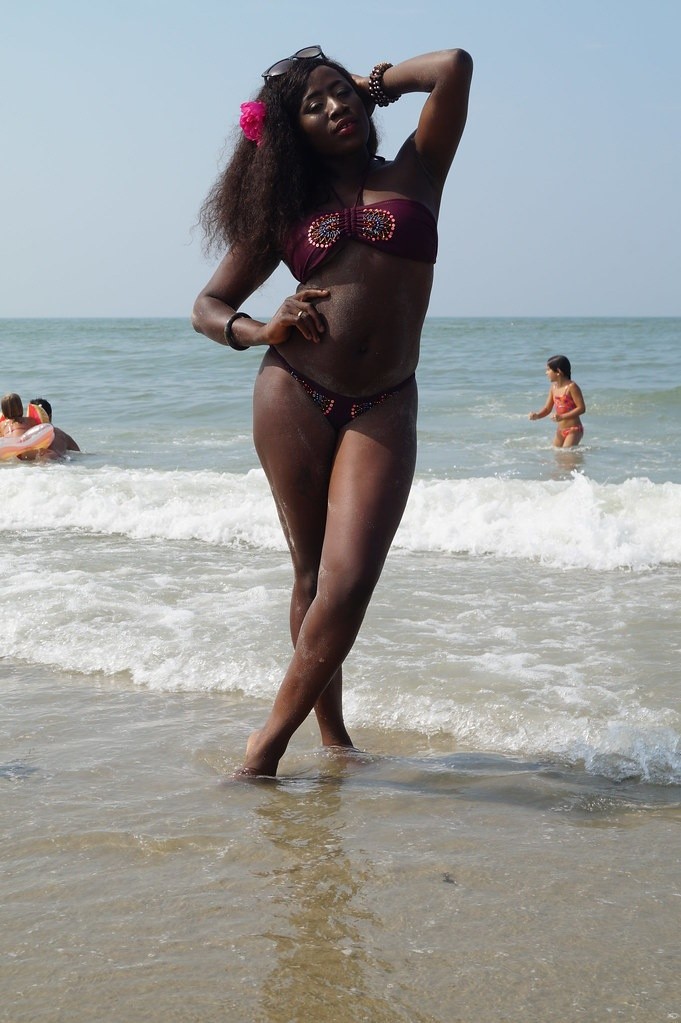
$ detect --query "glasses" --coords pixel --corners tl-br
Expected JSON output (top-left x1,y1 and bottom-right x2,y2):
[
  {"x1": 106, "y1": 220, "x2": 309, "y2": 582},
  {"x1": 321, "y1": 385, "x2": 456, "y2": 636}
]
[{"x1": 261, "y1": 44, "x2": 327, "y2": 81}]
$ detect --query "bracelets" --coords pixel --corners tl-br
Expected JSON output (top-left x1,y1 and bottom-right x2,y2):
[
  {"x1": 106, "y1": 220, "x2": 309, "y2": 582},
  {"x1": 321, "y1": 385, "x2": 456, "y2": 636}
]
[
  {"x1": 369, "y1": 63, "x2": 401, "y2": 107},
  {"x1": 223, "y1": 313, "x2": 252, "y2": 350}
]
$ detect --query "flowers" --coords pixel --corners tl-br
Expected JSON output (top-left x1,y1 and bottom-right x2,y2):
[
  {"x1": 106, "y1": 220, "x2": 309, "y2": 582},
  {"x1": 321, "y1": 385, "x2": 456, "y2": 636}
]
[{"x1": 239, "y1": 99, "x2": 268, "y2": 147}]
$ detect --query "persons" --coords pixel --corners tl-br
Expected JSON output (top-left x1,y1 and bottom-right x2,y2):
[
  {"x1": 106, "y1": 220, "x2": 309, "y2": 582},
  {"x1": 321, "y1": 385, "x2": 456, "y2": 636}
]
[
  {"x1": 0, "y1": 394, "x2": 80, "y2": 459},
  {"x1": 529, "y1": 355, "x2": 585, "y2": 447},
  {"x1": 189, "y1": 45, "x2": 476, "y2": 773}
]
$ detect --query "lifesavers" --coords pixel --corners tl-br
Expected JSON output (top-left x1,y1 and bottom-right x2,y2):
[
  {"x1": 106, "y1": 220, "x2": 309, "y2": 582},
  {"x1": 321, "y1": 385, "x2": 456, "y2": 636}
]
[{"x1": 0, "y1": 423, "x2": 55, "y2": 461}]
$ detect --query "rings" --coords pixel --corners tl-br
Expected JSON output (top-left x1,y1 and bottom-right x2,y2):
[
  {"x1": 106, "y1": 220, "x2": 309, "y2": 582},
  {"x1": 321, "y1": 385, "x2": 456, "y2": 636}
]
[{"x1": 297, "y1": 310, "x2": 303, "y2": 316}]
